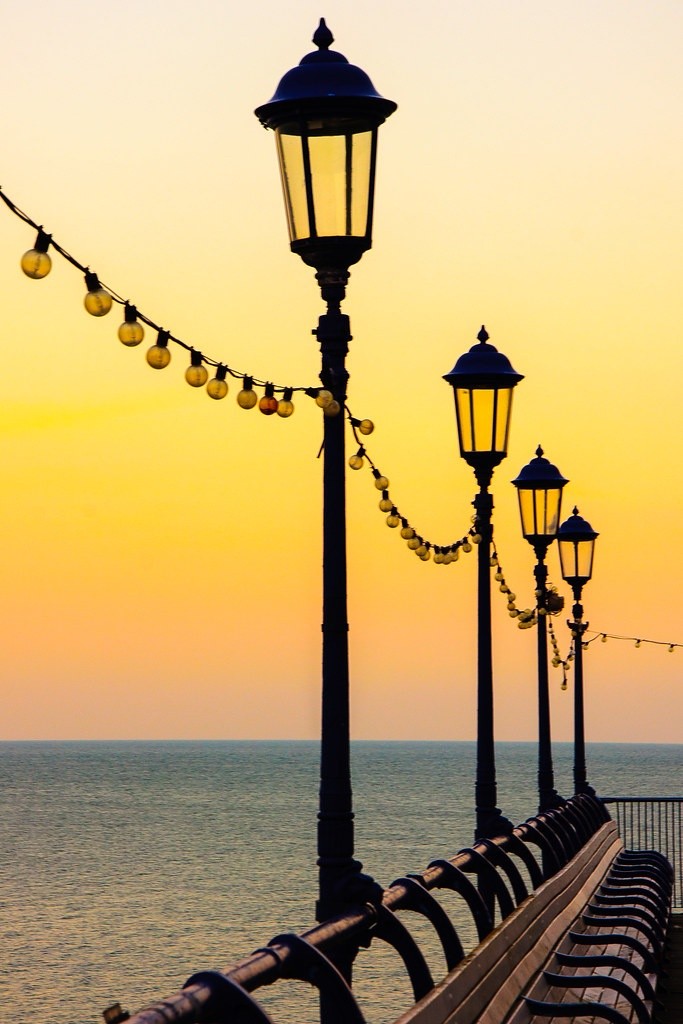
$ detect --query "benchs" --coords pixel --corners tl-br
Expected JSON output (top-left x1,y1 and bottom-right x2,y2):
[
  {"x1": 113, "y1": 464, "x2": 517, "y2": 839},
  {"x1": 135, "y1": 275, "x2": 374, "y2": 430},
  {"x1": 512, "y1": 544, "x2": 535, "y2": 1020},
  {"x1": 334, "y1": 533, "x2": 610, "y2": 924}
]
[{"x1": 103, "y1": 785, "x2": 676, "y2": 1024}]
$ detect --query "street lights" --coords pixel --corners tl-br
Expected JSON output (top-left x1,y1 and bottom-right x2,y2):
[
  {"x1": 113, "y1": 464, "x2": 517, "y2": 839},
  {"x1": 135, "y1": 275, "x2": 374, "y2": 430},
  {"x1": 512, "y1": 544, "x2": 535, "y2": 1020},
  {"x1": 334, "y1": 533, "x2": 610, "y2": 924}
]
[
  {"x1": 438, "y1": 325, "x2": 526, "y2": 921},
  {"x1": 507, "y1": 445, "x2": 574, "y2": 819},
  {"x1": 255, "y1": 14, "x2": 385, "y2": 938},
  {"x1": 550, "y1": 507, "x2": 608, "y2": 802}
]
